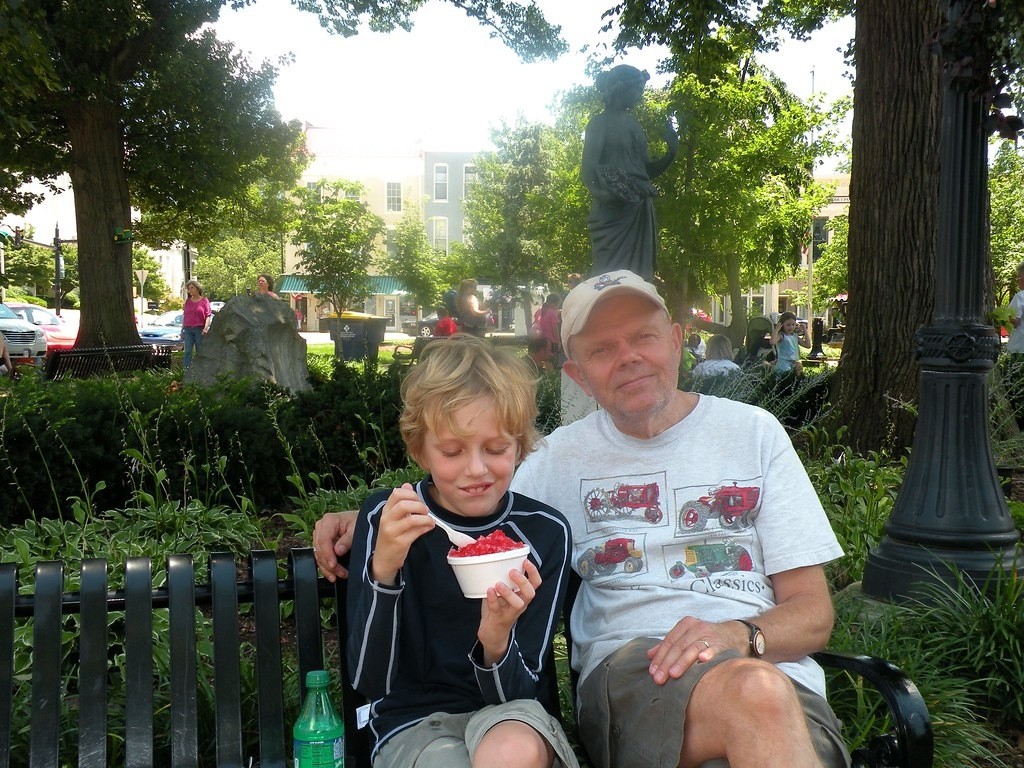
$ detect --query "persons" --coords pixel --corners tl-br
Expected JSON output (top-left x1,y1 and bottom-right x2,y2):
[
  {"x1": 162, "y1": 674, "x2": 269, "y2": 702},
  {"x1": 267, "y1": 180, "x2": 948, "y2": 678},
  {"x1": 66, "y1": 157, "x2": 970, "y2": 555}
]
[
  {"x1": 255, "y1": 275, "x2": 278, "y2": 299},
  {"x1": 522, "y1": 335, "x2": 553, "y2": 429},
  {"x1": 346, "y1": 332, "x2": 581, "y2": 768},
  {"x1": 456, "y1": 278, "x2": 491, "y2": 336},
  {"x1": 433, "y1": 305, "x2": 458, "y2": 335},
  {"x1": 682, "y1": 332, "x2": 742, "y2": 376},
  {"x1": 1006, "y1": 262, "x2": 1024, "y2": 379},
  {"x1": 295, "y1": 308, "x2": 302, "y2": 330},
  {"x1": 581, "y1": 64, "x2": 677, "y2": 285},
  {"x1": 312, "y1": 264, "x2": 852, "y2": 767},
  {"x1": 181, "y1": 280, "x2": 212, "y2": 382},
  {"x1": 533, "y1": 273, "x2": 585, "y2": 370},
  {"x1": 770, "y1": 311, "x2": 812, "y2": 372}
]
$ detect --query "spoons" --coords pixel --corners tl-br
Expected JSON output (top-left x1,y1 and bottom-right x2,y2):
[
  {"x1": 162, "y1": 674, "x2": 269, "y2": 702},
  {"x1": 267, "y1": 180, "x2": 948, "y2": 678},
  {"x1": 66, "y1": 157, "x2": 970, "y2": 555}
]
[{"x1": 427, "y1": 510, "x2": 476, "y2": 546}]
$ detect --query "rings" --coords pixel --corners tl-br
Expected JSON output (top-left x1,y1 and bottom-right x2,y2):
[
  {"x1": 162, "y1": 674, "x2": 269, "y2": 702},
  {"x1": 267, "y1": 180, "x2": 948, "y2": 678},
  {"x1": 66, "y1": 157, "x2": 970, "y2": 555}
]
[
  {"x1": 314, "y1": 548, "x2": 317, "y2": 553},
  {"x1": 698, "y1": 640, "x2": 709, "y2": 647}
]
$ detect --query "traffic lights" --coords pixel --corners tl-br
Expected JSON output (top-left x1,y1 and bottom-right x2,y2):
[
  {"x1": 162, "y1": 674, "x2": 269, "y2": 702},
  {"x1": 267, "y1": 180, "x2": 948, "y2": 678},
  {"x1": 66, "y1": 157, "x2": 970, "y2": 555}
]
[{"x1": 14, "y1": 227, "x2": 24, "y2": 246}]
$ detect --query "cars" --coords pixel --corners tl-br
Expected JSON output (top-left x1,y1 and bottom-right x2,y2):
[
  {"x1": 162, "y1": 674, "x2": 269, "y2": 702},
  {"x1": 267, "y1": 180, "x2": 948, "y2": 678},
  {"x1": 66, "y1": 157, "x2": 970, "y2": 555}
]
[
  {"x1": 402, "y1": 312, "x2": 458, "y2": 337},
  {"x1": 0, "y1": 301, "x2": 226, "y2": 356}
]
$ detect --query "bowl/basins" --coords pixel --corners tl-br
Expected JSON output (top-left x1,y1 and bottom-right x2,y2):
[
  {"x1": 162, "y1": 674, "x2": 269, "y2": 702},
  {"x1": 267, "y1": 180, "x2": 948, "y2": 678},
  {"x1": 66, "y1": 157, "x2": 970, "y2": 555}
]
[{"x1": 446, "y1": 544, "x2": 532, "y2": 600}]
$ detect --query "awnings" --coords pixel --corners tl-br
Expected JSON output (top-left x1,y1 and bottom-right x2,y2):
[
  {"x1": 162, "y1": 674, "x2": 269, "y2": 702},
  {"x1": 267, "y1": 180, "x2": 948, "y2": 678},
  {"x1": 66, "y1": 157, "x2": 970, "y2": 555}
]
[{"x1": 279, "y1": 275, "x2": 413, "y2": 292}]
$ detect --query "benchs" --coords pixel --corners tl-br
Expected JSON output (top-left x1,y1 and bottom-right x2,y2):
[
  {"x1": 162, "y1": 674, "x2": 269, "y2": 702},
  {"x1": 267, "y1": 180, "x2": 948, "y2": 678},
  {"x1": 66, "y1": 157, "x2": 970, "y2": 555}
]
[
  {"x1": 392, "y1": 335, "x2": 464, "y2": 366},
  {"x1": 8, "y1": 343, "x2": 176, "y2": 381},
  {"x1": 0, "y1": 548, "x2": 934, "y2": 768}
]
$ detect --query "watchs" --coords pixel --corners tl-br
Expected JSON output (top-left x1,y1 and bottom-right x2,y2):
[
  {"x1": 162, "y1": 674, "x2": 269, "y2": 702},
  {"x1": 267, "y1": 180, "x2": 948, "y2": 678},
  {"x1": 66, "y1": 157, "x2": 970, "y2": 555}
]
[{"x1": 733, "y1": 619, "x2": 766, "y2": 660}]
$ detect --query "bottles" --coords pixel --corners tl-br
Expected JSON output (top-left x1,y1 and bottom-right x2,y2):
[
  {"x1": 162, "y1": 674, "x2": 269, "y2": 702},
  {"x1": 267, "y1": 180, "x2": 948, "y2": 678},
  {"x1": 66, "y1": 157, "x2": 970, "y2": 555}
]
[
  {"x1": 489, "y1": 314, "x2": 495, "y2": 324},
  {"x1": 294, "y1": 671, "x2": 345, "y2": 767}
]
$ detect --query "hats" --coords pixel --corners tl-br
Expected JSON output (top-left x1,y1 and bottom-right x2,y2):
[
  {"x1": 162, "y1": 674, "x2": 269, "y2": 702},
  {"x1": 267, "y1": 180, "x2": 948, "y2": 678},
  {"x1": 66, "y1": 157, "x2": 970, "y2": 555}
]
[{"x1": 561, "y1": 269, "x2": 671, "y2": 361}]
